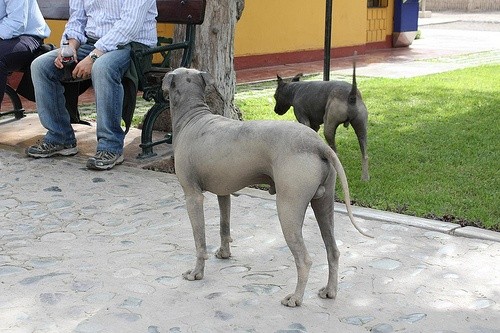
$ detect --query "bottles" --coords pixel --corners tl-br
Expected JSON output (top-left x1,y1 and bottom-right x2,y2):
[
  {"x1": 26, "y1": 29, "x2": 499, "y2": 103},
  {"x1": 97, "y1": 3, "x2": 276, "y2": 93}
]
[{"x1": 61, "y1": 40, "x2": 76, "y2": 81}]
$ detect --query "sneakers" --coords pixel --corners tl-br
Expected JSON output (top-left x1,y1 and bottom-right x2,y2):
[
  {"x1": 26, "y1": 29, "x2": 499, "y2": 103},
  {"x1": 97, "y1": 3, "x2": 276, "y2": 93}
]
[
  {"x1": 25, "y1": 138, "x2": 79, "y2": 159},
  {"x1": 86, "y1": 149, "x2": 125, "y2": 170}
]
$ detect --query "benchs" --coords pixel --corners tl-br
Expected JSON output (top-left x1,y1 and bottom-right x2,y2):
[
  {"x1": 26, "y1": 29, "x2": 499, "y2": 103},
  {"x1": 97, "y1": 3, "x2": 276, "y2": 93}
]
[{"x1": 0, "y1": 0, "x2": 206, "y2": 159}]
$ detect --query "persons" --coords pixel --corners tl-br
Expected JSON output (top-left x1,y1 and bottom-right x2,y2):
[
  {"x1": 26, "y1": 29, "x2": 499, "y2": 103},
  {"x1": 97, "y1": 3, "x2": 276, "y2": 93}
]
[
  {"x1": 0, "y1": 0, "x2": 51, "y2": 112},
  {"x1": 27, "y1": 0, "x2": 158, "y2": 170}
]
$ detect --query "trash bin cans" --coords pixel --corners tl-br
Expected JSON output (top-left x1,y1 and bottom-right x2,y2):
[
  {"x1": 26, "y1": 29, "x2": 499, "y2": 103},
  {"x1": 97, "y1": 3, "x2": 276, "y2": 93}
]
[{"x1": 393, "y1": 0, "x2": 418, "y2": 48}]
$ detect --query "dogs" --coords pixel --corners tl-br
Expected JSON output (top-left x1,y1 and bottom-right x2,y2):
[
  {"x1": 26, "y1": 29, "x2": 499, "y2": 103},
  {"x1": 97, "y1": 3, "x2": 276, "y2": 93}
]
[
  {"x1": 273, "y1": 60, "x2": 370, "y2": 183},
  {"x1": 161, "y1": 67, "x2": 375, "y2": 307}
]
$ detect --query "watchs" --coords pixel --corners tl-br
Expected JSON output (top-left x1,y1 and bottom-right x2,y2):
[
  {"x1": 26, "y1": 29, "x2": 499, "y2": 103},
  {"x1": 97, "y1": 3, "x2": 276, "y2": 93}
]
[{"x1": 88, "y1": 52, "x2": 100, "y2": 63}]
